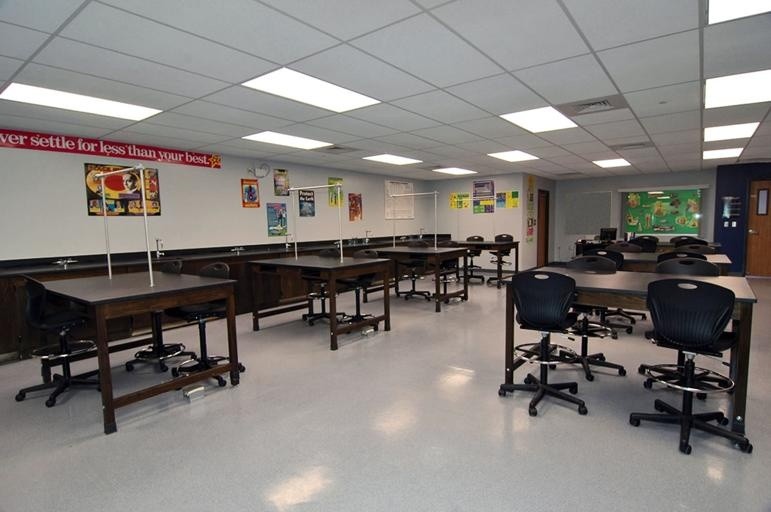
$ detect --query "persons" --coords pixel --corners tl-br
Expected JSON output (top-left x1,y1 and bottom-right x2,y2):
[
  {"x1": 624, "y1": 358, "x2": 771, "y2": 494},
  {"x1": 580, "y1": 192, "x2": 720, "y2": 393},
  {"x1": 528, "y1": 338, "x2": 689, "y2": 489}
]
[{"x1": 123, "y1": 173, "x2": 139, "y2": 195}]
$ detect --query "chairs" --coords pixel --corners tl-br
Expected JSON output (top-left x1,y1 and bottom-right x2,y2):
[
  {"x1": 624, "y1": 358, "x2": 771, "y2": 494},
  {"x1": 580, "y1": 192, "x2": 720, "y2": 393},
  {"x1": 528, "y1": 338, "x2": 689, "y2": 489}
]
[
  {"x1": 378, "y1": 232, "x2": 518, "y2": 314},
  {"x1": 334, "y1": 249, "x2": 379, "y2": 334},
  {"x1": 566, "y1": 234, "x2": 754, "y2": 385},
  {"x1": 171, "y1": 261, "x2": 245, "y2": 389},
  {"x1": 124, "y1": 260, "x2": 197, "y2": 374},
  {"x1": 499, "y1": 270, "x2": 587, "y2": 419},
  {"x1": 629, "y1": 277, "x2": 753, "y2": 458},
  {"x1": 14, "y1": 272, "x2": 107, "y2": 408},
  {"x1": 300, "y1": 247, "x2": 348, "y2": 327}
]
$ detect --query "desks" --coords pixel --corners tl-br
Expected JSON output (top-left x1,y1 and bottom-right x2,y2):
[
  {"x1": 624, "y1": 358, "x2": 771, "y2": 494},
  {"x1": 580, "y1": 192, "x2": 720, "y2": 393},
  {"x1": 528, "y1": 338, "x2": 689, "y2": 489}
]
[
  {"x1": 245, "y1": 254, "x2": 391, "y2": 351},
  {"x1": 40, "y1": 270, "x2": 240, "y2": 435},
  {"x1": 499, "y1": 266, "x2": 756, "y2": 436}
]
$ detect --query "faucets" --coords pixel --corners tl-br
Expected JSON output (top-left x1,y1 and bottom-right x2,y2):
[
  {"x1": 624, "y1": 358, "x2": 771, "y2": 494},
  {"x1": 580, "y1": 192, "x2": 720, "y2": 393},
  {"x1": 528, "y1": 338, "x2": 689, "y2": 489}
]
[
  {"x1": 157, "y1": 251, "x2": 165, "y2": 259},
  {"x1": 230, "y1": 246, "x2": 245, "y2": 256},
  {"x1": 284, "y1": 243, "x2": 291, "y2": 249},
  {"x1": 364, "y1": 237, "x2": 372, "y2": 243},
  {"x1": 60, "y1": 257, "x2": 68, "y2": 270},
  {"x1": 334, "y1": 240, "x2": 341, "y2": 248},
  {"x1": 400, "y1": 236, "x2": 407, "y2": 242},
  {"x1": 418, "y1": 227, "x2": 425, "y2": 240}
]
